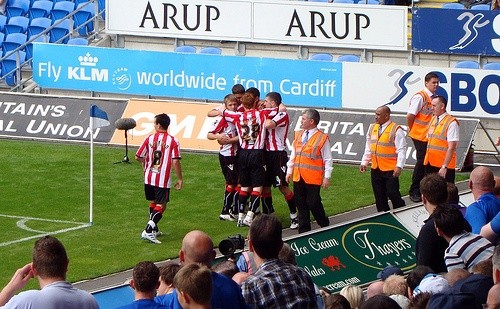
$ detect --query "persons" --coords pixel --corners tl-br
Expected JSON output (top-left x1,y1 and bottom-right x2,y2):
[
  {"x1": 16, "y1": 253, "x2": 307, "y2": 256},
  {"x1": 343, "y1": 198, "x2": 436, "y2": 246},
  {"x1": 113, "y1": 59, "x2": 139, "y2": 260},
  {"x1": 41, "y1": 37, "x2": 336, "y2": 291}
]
[
  {"x1": 207, "y1": 84, "x2": 299, "y2": 229},
  {"x1": 0, "y1": 235, "x2": 100, "y2": 309},
  {"x1": 285, "y1": 108, "x2": 334, "y2": 233},
  {"x1": 134, "y1": 113, "x2": 183, "y2": 244},
  {"x1": 359, "y1": 106, "x2": 408, "y2": 213},
  {"x1": 405, "y1": 72, "x2": 440, "y2": 203},
  {"x1": 422, "y1": 96, "x2": 460, "y2": 184},
  {"x1": 112, "y1": 166, "x2": 500, "y2": 309}
]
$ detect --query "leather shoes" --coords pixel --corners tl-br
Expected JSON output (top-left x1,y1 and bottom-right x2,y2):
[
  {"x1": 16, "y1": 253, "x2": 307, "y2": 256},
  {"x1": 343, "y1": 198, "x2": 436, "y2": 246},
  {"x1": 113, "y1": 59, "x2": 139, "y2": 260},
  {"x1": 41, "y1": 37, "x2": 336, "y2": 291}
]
[{"x1": 409, "y1": 195, "x2": 422, "y2": 202}]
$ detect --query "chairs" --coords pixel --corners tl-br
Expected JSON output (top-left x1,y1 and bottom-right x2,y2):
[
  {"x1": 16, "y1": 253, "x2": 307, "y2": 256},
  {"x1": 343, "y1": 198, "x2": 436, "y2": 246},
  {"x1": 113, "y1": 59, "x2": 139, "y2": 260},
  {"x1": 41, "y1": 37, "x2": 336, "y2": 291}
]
[
  {"x1": 311, "y1": 54, "x2": 333, "y2": 61},
  {"x1": 176, "y1": 46, "x2": 196, "y2": 53},
  {"x1": 484, "y1": 62, "x2": 500, "y2": 70},
  {"x1": 471, "y1": 4, "x2": 490, "y2": 10},
  {"x1": 338, "y1": 55, "x2": 359, "y2": 62},
  {"x1": 308, "y1": 0, "x2": 380, "y2": 4},
  {"x1": 0, "y1": 0, "x2": 105, "y2": 86},
  {"x1": 443, "y1": 3, "x2": 464, "y2": 9},
  {"x1": 455, "y1": 60, "x2": 479, "y2": 68},
  {"x1": 200, "y1": 48, "x2": 222, "y2": 54}
]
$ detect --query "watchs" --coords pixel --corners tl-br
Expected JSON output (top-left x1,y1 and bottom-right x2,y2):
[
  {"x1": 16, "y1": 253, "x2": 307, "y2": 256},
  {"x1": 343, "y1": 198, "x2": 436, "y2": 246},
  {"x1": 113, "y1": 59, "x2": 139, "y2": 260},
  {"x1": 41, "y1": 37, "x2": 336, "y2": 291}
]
[{"x1": 442, "y1": 165, "x2": 448, "y2": 169}]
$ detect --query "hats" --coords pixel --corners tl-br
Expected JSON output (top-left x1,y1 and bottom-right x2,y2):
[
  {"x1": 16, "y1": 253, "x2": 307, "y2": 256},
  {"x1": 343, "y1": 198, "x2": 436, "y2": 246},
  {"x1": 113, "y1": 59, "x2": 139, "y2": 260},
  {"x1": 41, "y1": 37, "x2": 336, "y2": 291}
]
[{"x1": 376, "y1": 265, "x2": 404, "y2": 279}]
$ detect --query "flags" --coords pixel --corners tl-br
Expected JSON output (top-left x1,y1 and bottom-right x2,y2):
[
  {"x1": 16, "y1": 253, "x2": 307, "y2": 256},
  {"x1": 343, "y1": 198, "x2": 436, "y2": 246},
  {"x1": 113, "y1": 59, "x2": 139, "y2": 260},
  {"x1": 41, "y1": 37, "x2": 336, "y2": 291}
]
[{"x1": 90, "y1": 105, "x2": 111, "y2": 130}]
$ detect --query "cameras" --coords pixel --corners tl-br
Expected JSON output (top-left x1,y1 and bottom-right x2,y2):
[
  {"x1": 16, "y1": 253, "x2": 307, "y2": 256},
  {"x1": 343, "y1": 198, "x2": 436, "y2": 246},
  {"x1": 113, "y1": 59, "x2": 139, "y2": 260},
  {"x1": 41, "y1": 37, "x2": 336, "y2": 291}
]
[{"x1": 219, "y1": 233, "x2": 245, "y2": 257}]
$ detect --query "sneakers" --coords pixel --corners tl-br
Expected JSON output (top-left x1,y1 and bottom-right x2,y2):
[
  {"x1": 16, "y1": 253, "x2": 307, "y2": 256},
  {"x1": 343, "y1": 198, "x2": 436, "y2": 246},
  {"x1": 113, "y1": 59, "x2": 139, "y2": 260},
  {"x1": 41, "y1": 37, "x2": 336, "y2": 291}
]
[
  {"x1": 237, "y1": 212, "x2": 246, "y2": 227},
  {"x1": 288, "y1": 212, "x2": 299, "y2": 229},
  {"x1": 229, "y1": 213, "x2": 238, "y2": 221},
  {"x1": 219, "y1": 213, "x2": 229, "y2": 220},
  {"x1": 141, "y1": 230, "x2": 162, "y2": 245},
  {"x1": 155, "y1": 231, "x2": 163, "y2": 236},
  {"x1": 243, "y1": 211, "x2": 254, "y2": 227}
]
